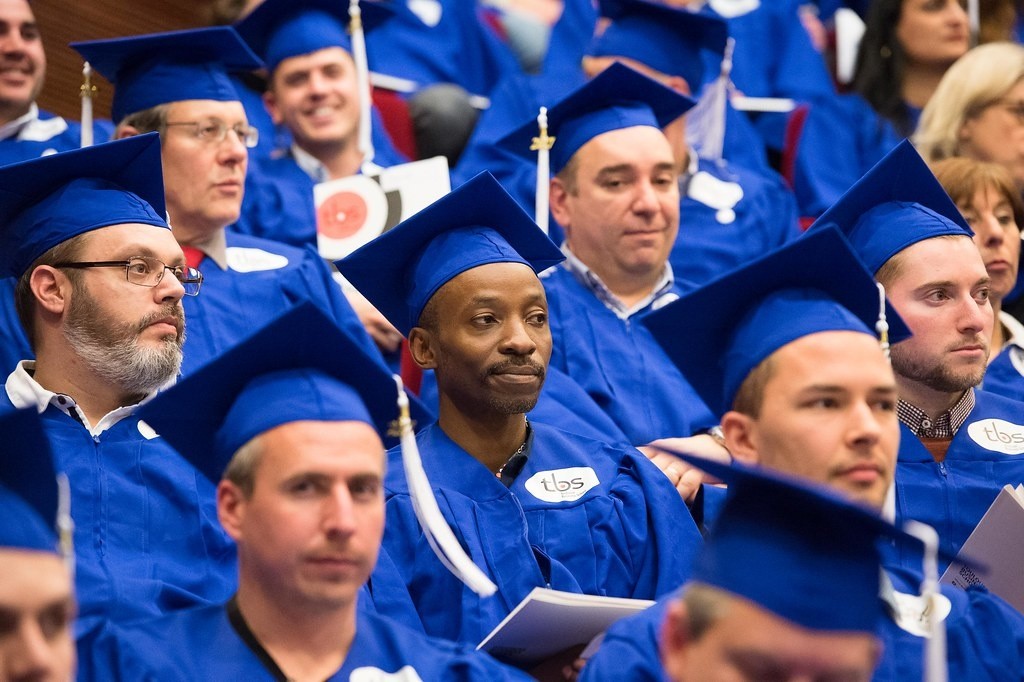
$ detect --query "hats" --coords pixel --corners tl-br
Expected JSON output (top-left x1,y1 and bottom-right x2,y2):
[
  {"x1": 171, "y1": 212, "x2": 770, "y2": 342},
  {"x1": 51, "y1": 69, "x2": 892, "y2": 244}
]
[
  {"x1": 70, "y1": 25, "x2": 265, "y2": 150},
  {"x1": 583, "y1": 0, "x2": 735, "y2": 162},
  {"x1": 0, "y1": 131, "x2": 175, "y2": 279},
  {"x1": 494, "y1": 60, "x2": 698, "y2": 237},
  {"x1": 1, "y1": 403, "x2": 75, "y2": 568},
  {"x1": 639, "y1": 223, "x2": 912, "y2": 523},
  {"x1": 135, "y1": 299, "x2": 500, "y2": 601},
  {"x1": 334, "y1": 171, "x2": 566, "y2": 339},
  {"x1": 796, "y1": 138, "x2": 976, "y2": 277},
  {"x1": 237, "y1": 0, "x2": 395, "y2": 162},
  {"x1": 642, "y1": 438, "x2": 950, "y2": 682}
]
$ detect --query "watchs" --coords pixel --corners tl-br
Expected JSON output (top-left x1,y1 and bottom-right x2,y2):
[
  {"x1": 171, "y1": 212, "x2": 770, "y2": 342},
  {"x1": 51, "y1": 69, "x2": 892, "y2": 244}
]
[{"x1": 708, "y1": 425, "x2": 725, "y2": 444}]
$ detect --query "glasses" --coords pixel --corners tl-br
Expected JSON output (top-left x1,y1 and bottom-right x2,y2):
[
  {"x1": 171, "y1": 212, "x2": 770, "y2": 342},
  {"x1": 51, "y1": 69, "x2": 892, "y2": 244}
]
[
  {"x1": 137, "y1": 118, "x2": 261, "y2": 151},
  {"x1": 49, "y1": 256, "x2": 204, "y2": 296}
]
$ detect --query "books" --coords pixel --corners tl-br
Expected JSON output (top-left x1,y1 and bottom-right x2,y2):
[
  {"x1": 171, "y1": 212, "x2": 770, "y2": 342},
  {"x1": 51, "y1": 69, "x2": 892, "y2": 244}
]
[{"x1": 475, "y1": 586, "x2": 657, "y2": 672}]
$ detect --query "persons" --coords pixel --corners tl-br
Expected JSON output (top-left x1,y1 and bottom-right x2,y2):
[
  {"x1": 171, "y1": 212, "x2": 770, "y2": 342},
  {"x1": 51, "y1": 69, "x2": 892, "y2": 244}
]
[
  {"x1": 68, "y1": 25, "x2": 394, "y2": 381},
  {"x1": 804, "y1": 136, "x2": 1024, "y2": 580},
  {"x1": 225, "y1": 0, "x2": 410, "y2": 252},
  {"x1": 494, "y1": 60, "x2": 720, "y2": 447},
  {"x1": 498, "y1": 0, "x2": 802, "y2": 286},
  {"x1": 364, "y1": 0, "x2": 525, "y2": 168},
  {"x1": 577, "y1": 224, "x2": 1024, "y2": 682},
  {"x1": 0, "y1": 0, "x2": 116, "y2": 385},
  {"x1": 908, "y1": 41, "x2": 1024, "y2": 192},
  {"x1": 575, "y1": 444, "x2": 992, "y2": 682},
  {"x1": 333, "y1": 169, "x2": 705, "y2": 682},
  {"x1": 540, "y1": 0, "x2": 834, "y2": 285},
  {"x1": 76, "y1": 297, "x2": 539, "y2": 682},
  {"x1": 793, "y1": 0, "x2": 971, "y2": 222},
  {"x1": 0, "y1": 131, "x2": 238, "y2": 626},
  {"x1": 927, "y1": 157, "x2": 1024, "y2": 402},
  {"x1": 0, "y1": 404, "x2": 78, "y2": 682},
  {"x1": 938, "y1": 483, "x2": 1024, "y2": 615}
]
